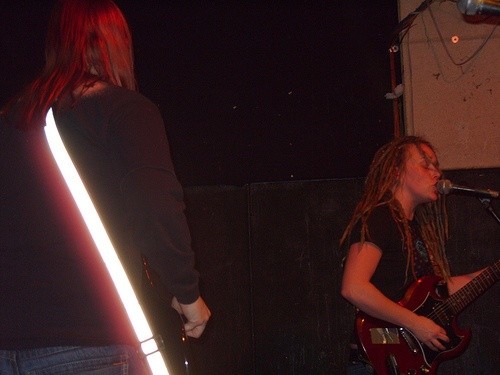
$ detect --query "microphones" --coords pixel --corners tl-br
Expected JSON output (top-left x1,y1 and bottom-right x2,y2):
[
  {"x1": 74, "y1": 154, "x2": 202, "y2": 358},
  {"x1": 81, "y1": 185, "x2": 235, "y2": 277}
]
[
  {"x1": 437, "y1": 180, "x2": 500, "y2": 198},
  {"x1": 458, "y1": 0, "x2": 500, "y2": 16}
]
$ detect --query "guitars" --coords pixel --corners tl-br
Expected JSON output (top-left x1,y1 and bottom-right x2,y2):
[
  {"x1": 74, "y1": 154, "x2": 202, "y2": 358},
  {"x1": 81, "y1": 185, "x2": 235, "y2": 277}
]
[
  {"x1": 140, "y1": 258, "x2": 193, "y2": 375},
  {"x1": 356, "y1": 259, "x2": 500, "y2": 375}
]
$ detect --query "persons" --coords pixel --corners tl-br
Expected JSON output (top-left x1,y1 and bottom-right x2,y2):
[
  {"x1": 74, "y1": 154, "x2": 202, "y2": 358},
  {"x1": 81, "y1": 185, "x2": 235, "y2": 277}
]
[
  {"x1": 338, "y1": 135, "x2": 448, "y2": 375},
  {"x1": 0, "y1": 0, "x2": 212, "y2": 375}
]
